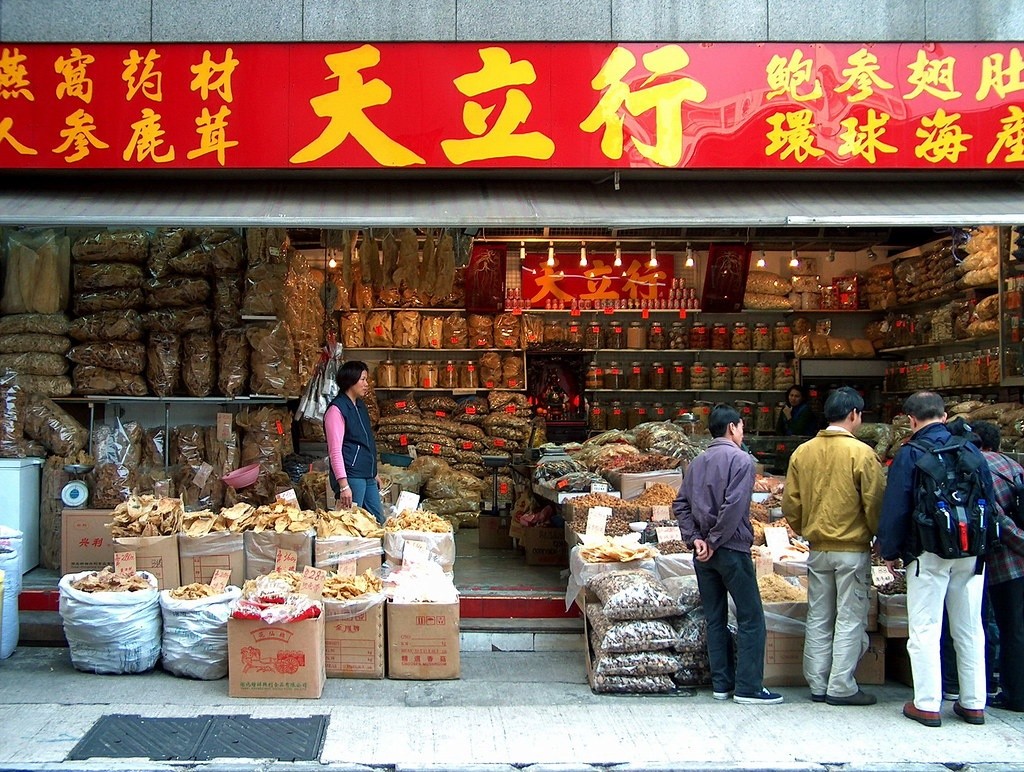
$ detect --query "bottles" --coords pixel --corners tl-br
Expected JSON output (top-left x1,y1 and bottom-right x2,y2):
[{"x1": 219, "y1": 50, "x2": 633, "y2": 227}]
[
  {"x1": 377, "y1": 359, "x2": 481, "y2": 388},
  {"x1": 883, "y1": 346, "x2": 1024, "y2": 425},
  {"x1": 542, "y1": 318, "x2": 793, "y2": 444}
]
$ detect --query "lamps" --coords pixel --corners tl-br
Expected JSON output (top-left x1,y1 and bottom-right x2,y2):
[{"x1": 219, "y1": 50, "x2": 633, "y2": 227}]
[
  {"x1": 685, "y1": 240, "x2": 694, "y2": 268},
  {"x1": 547, "y1": 241, "x2": 555, "y2": 266},
  {"x1": 650, "y1": 241, "x2": 657, "y2": 267},
  {"x1": 614, "y1": 241, "x2": 622, "y2": 266},
  {"x1": 579, "y1": 241, "x2": 587, "y2": 266},
  {"x1": 461, "y1": 228, "x2": 480, "y2": 237},
  {"x1": 825, "y1": 248, "x2": 836, "y2": 263},
  {"x1": 866, "y1": 244, "x2": 878, "y2": 263},
  {"x1": 788, "y1": 242, "x2": 800, "y2": 267},
  {"x1": 756, "y1": 242, "x2": 767, "y2": 267},
  {"x1": 519, "y1": 241, "x2": 527, "y2": 259}
]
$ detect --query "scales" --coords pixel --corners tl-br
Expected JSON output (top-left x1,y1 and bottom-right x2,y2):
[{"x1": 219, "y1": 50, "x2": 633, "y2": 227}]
[
  {"x1": 535, "y1": 445, "x2": 572, "y2": 466},
  {"x1": 59, "y1": 464, "x2": 95, "y2": 510},
  {"x1": 480, "y1": 455, "x2": 512, "y2": 514}
]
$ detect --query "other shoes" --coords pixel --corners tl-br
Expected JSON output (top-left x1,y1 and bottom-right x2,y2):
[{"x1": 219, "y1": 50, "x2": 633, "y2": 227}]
[
  {"x1": 988, "y1": 697, "x2": 1013, "y2": 711},
  {"x1": 986, "y1": 686, "x2": 1000, "y2": 698},
  {"x1": 826, "y1": 689, "x2": 878, "y2": 705},
  {"x1": 943, "y1": 684, "x2": 960, "y2": 700},
  {"x1": 812, "y1": 693, "x2": 827, "y2": 703}
]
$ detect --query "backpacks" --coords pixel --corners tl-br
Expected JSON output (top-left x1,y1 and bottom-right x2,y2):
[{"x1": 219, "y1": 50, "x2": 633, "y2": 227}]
[
  {"x1": 908, "y1": 434, "x2": 999, "y2": 559},
  {"x1": 986, "y1": 453, "x2": 1024, "y2": 531}
]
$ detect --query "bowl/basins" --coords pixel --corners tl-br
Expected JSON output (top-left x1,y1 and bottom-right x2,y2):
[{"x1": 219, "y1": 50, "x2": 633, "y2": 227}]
[
  {"x1": 380, "y1": 452, "x2": 415, "y2": 468},
  {"x1": 221, "y1": 464, "x2": 261, "y2": 488},
  {"x1": 629, "y1": 522, "x2": 648, "y2": 533}
]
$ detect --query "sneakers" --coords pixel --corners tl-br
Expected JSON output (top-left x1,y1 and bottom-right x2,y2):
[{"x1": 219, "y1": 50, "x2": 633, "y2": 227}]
[
  {"x1": 734, "y1": 687, "x2": 784, "y2": 705},
  {"x1": 712, "y1": 689, "x2": 735, "y2": 700}
]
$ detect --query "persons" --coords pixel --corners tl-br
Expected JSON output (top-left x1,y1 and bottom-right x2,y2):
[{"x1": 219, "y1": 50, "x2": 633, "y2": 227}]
[
  {"x1": 672, "y1": 404, "x2": 784, "y2": 704},
  {"x1": 781, "y1": 387, "x2": 887, "y2": 705},
  {"x1": 540, "y1": 368, "x2": 569, "y2": 406},
  {"x1": 969, "y1": 419, "x2": 1024, "y2": 712},
  {"x1": 876, "y1": 390, "x2": 998, "y2": 727},
  {"x1": 776, "y1": 385, "x2": 816, "y2": 476},
  {"x1": 322, "y1": 360, "x2": 386, "y2": 526},
  {"x1": 940, "y1": 416, "x2": 997, "y2": 701}
]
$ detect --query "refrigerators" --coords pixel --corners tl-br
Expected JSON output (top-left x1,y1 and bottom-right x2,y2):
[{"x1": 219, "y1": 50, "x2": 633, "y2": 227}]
[{"x1": 0, "y1": 457, "x2": 45, "y2": 575}]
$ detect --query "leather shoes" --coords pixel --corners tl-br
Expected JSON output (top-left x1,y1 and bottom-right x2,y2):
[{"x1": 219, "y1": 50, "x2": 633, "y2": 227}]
[
  {"x1": 903, "y1": 701, "x2": 941, "y2": 728},
  {"x1": 953, "y1": 700, "x2": 985, "y2": 725}
]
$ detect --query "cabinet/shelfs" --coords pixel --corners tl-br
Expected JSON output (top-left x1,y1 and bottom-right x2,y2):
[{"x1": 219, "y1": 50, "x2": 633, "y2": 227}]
[
  {"x1": 336, "y1": 283, "x2": 1024, "y2": 432},
  {"x1": 46, "y1": 314, "x2": 299, "y2": 467}
]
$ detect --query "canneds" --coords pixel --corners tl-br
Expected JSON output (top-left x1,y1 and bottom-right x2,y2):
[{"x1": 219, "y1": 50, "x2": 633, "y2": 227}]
[
  {"x1": 570, "y1": 298, "x2": 667, "y2": 310},
  {"x1": 545, "y1": 299, "x2": 565, "y2": 310},
  {"x1": 667, "y1": 278, "x2": 700, "y2": 310},
  {"x1": 504, "y1": 287, "x2": 531, "y2": 309}
]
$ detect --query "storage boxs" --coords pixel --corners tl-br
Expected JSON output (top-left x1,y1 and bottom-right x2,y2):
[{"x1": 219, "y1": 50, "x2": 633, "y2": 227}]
[
  {"x1": 477, "y1": 512, "x2": 512, "y2": 549},
  {"x1": 61, "y1": 475, "x2": 461, "y2": 699},
  {"x1": 819, "y1": 275, "x2": 860, "y2": 310},
  {"x1": 509, "y1": 456, "x2": 913, "y2": 687}
]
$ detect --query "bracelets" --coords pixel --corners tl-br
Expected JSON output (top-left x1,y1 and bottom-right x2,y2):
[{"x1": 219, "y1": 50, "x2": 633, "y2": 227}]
[{"x1": 340, "y1": 484, "x2": 349, "y2": 492}]
